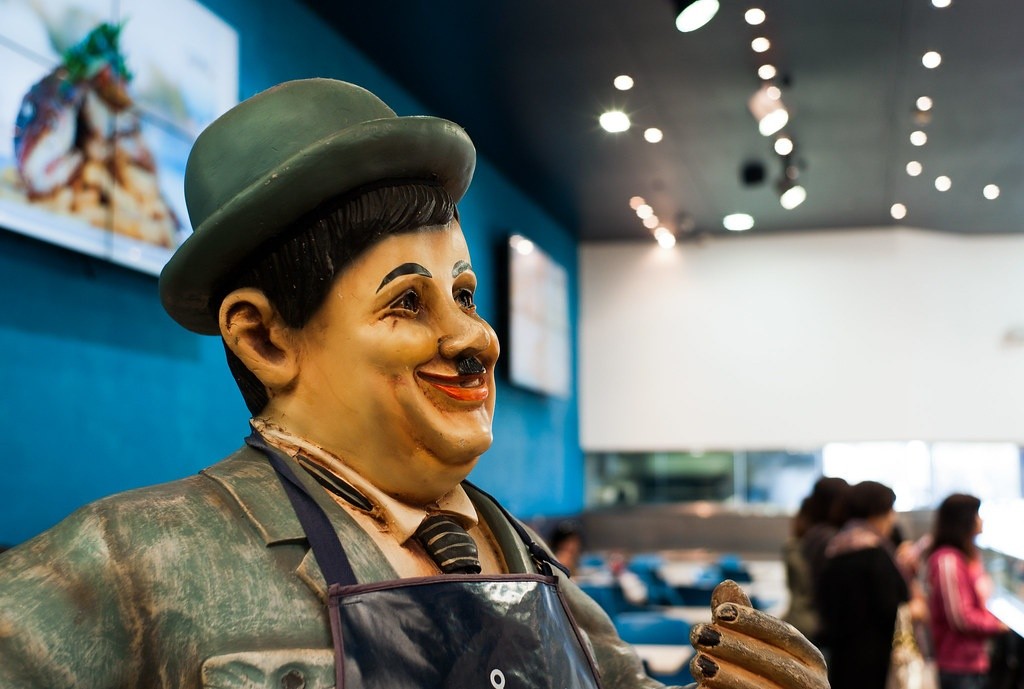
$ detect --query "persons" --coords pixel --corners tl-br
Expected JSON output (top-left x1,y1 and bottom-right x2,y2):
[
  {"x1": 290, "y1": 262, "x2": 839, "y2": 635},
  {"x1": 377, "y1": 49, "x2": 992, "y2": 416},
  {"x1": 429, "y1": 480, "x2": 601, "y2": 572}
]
[
  {"x1": 548, "y1": 520, "x2": 649, "y2": 601},
  {"x1": 924, "y1": 493, "x2": 1012, "y2": 689},
  {"x1": 0, "y1": 77, "x2": 830, "y2": 688},
  {"x1": 778, "y1": 476, "x2": 932, "y2": 689}
]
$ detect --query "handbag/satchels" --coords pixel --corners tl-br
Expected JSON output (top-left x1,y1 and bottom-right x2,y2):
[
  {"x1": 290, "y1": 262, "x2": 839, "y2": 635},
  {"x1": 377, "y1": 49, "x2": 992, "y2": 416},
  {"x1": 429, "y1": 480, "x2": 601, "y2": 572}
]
[{"x1": 890, "y1": 605, "x2": 928, "y2": 689}]
